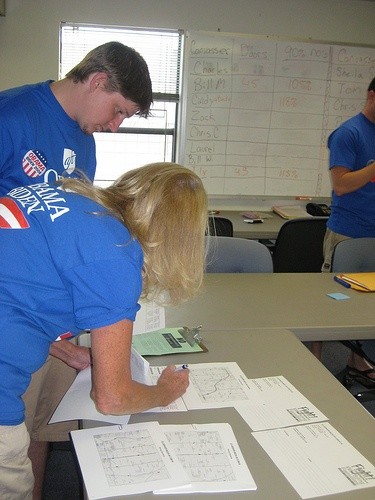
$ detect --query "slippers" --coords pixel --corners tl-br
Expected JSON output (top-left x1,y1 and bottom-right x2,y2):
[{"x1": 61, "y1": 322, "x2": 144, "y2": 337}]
[{"x1": 346, "y1": 363, "x2": 375, "y2": 382}]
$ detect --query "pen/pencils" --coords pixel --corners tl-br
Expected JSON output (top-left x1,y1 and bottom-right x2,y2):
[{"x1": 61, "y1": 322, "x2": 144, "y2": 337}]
[
  {"x1": 244, "y1": 219, "x2": 263, "y2": 224},
  {"x1": 175, "y1": 364, "x2": 188, "y2": 371},
  {"x1": 334, "y1": 276, "x2": 350, "y2": 288},
  {"x1": 209, "y1": 211, "x2": 219, "y2": 215}
]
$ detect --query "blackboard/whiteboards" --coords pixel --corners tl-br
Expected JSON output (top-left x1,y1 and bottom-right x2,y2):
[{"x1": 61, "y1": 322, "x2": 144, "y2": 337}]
[{"x1": 180, "y1": 28, "x2": 375, "y2": 202}]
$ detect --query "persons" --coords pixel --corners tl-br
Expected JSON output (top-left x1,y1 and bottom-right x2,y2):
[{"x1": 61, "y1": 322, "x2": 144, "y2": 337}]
[
  {"x1": 0, "y1": 41, "x2": 153, "y2": 500},
  {"x1": 0, "y1": 162, "x2": 226, "y2": 500},
  {"x1": 312, "y1": 78, "x2": 375, "y2": 383}
]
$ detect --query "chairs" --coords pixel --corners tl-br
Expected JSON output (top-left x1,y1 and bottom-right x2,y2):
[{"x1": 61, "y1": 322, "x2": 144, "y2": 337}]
[
  {"x1": 208, "y1": 217, "x2": 233, "y2": 237},
  {"x1": 272, "y1": 219, "x2": 327, "y2": 273},
  {"x1": 204, "y1": 235, "x2": 274, "y2": 273},
  {"x1": 331, "y1": 238, "x2": 375, "y2": 365}
]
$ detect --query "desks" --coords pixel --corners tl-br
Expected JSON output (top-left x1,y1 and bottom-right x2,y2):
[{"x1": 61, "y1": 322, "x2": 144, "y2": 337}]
[
  {"x1": 272, "y1": 205, "x2": 330, "y2": 220},
  {"x1": 165, "y1": 273, "x2": 375, "y2": 341},
  {"x1": 78, "y1": 330, "x2": 375, "y2": 499},
  {"x1": 208, "y1": 210, "x2": 289, "y2": 239}
]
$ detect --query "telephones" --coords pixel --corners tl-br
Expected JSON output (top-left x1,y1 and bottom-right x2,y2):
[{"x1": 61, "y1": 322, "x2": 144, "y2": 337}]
[{"x1": 306, "y1": 203, "x2": 331, "y2": 216}]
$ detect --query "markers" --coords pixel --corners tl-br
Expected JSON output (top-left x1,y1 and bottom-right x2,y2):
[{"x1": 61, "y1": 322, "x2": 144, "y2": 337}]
[{"x1": 296, "y1": 197, "x2": 311, "y2": 200}]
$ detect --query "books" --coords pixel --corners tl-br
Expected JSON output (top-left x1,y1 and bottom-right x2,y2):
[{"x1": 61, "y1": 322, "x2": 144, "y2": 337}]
[{"x1": 274, "y1": 206, "x2": 311, "y2": 219}]
